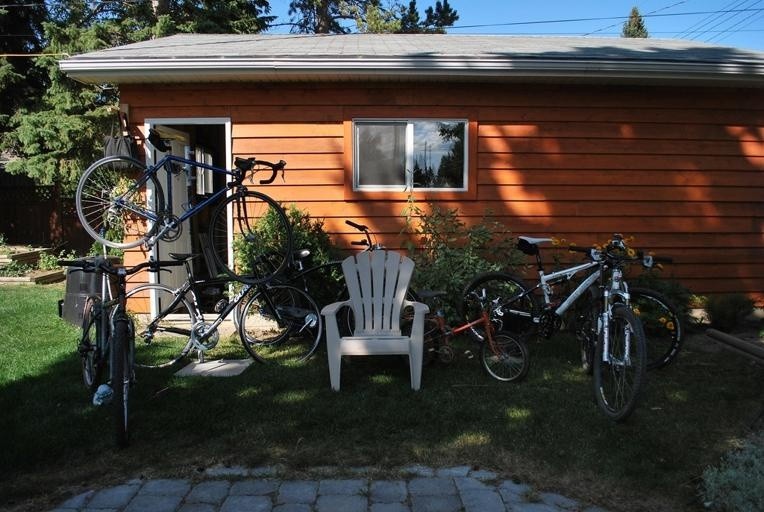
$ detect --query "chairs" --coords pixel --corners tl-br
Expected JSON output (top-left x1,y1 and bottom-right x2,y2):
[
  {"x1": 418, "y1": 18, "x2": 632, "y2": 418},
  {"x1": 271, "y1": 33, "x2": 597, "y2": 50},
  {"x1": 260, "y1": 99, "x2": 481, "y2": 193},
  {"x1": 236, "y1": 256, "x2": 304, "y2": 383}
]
[{"x1": 320, "y1": 250, "x2": 432, "y2": 392}]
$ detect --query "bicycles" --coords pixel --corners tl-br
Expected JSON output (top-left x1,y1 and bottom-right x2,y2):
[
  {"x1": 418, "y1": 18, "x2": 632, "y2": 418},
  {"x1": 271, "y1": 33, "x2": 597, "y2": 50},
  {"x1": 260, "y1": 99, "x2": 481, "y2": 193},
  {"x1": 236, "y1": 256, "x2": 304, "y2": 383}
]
[{"x1": 393, "y1": 287, "x2": 531, "y2": 384}]
